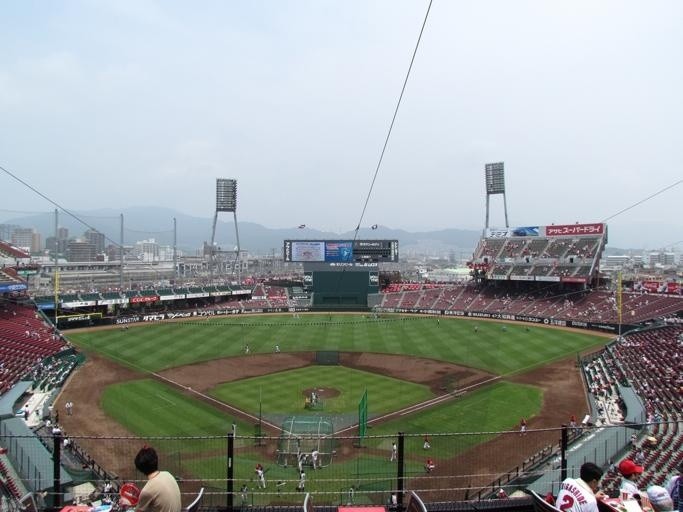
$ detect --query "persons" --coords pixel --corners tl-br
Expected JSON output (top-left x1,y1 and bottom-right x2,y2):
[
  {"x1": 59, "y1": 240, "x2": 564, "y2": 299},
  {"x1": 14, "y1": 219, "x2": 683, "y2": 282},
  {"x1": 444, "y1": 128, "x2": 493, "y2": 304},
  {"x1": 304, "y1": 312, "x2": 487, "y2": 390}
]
[
  {"x1": 346, "y1": 484, "x2": 355, "y2": 505},
  {"x1": 311, "y1": 449, "x2": 322, "y2": 470},
  {"x1": 555, "y1": 462, "x2": 606, "y2": 512},
  {"x1": 0, "y1": 307, "x2": 74, "y2": 448},
  {"x1": 202, "y1": 287, "x2": 299, "y2": 312},
  {"x1": 255, "y1": 463, "x2": 265, "y2": 488},
  {"x1": 519, "y1": 417, "x2": 529, "y2": 436},
  {"x1": 388, "y1": 440, "x2": 396, "y2": 461},
  {"x1": 69, "y1": 478, "x2": 132, "y2": 512},
  {"x1": 479, "y1": 238, "x2": 597, "y2": 280},
  {"x1": 132, "y1": 445, "x2": 183, "y2": 511},
  {"x1": 383, "y1": 281, "x2": 682, "y2": 322},
  {"x1": 570, "y1": 322, "x2": 683, "y2": 512},
  {"x1": 296, "y1": 469, "x2": 305, "y2": 492},
  {"x1": 240, "y1": 483, "x2": 247, "y2": 503}
]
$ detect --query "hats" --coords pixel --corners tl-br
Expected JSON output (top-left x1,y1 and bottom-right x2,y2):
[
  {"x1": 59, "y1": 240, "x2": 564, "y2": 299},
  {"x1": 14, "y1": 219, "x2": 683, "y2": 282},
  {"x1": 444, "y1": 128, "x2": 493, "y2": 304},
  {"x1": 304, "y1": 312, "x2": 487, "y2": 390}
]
[
  {"x1": 645, "y1": 484, "x2": 671, "y2": 502},
  {"x1": 616, "y1": 458, "x2": 645, "y2": 475}
]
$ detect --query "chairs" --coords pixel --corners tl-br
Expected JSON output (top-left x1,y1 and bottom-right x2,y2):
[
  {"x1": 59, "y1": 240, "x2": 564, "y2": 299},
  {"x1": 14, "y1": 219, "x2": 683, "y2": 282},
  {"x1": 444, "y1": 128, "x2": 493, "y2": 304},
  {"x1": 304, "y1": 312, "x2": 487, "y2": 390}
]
[
  {"x1": 381, "y1": 282, "x2": 443, "y2": 293},
  {"x1": 583, "y1": 324, "x2": 683, "y2": 512},
  {"x1": 0, "y1": 303, "x2": 65, "y2": 395}
]
